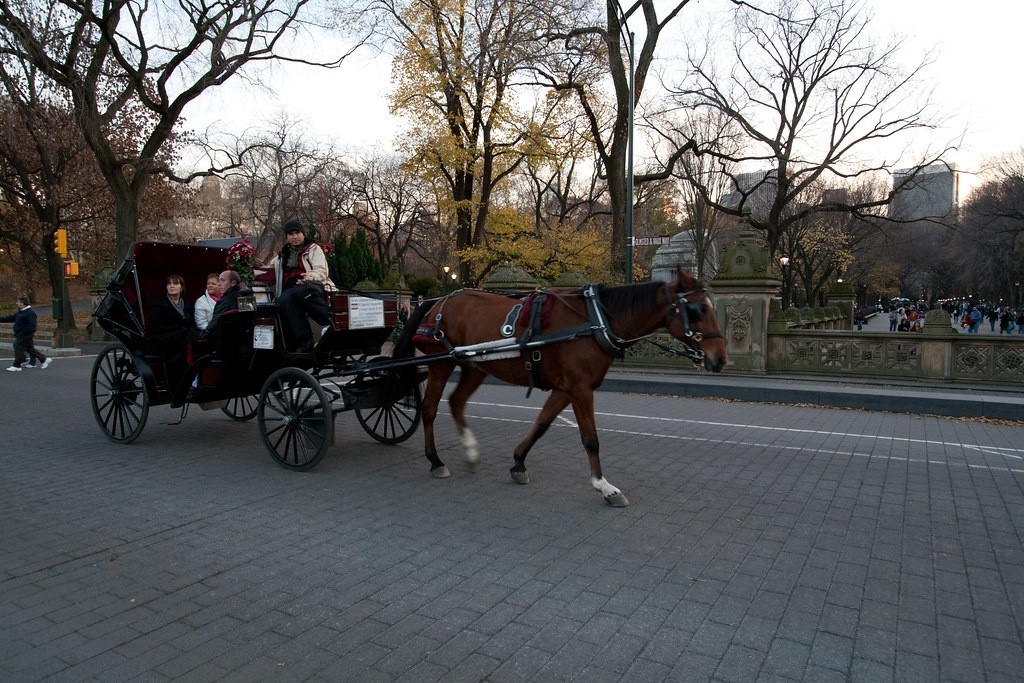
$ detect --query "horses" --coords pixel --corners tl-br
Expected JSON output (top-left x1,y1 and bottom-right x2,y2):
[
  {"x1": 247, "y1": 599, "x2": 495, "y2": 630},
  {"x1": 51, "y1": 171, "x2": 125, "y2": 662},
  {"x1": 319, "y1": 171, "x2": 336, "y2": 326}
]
[{"x1": 391, "y1": 266, "x2": 727, "y2": 508}]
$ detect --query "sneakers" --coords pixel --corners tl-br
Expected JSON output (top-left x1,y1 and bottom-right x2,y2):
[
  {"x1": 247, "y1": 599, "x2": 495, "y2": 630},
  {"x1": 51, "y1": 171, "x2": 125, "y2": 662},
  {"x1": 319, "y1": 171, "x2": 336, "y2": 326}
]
[
  {"x1": 21, "y1": 361, "x2": 28, "y2": 366},
  {"x1": 41, "y1": 358, "x2": 52, "y2": 369},
  {"x1": 6, "y1": 366, "x2": 22, "y2": 371},
  {"x1": 26, "y1": 364, "x2": 37, "y2": 368}
]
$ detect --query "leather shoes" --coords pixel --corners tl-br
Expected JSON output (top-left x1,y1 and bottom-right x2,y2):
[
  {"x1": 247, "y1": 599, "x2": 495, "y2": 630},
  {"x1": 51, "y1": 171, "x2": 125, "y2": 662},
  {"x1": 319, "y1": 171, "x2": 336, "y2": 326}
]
[{"x1": 292, "y1": 340, "x2": 314, "y2": 352}]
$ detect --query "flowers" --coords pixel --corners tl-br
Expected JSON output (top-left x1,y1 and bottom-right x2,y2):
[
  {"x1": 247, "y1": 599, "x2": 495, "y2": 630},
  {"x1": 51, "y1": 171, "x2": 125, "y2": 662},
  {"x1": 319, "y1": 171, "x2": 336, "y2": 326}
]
[
  {"x1": 318, "y1": 242, "x2": 333, "y2": 259},
  {"x1": 227, "y1": 238, "x2": 258, "y2": 288}
]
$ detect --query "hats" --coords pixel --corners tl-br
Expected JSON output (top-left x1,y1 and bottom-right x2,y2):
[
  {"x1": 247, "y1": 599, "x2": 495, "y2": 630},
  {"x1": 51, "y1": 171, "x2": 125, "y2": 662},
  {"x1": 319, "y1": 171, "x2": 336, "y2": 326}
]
[{"x1": 284, "y1": 221, "x2": 304, "y2": 235}]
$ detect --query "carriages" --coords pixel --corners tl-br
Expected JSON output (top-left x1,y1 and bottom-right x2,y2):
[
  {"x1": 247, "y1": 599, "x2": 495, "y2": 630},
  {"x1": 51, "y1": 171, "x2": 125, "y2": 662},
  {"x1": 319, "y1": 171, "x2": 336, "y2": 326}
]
[{"x1": 88, "y1": 239, "x2": 729, "y2": 509}]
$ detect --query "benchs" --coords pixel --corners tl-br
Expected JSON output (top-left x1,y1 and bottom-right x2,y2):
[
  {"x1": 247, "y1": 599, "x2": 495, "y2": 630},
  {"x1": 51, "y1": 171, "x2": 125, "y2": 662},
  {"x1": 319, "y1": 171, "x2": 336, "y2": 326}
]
[{"x1": 151, "y1": 285, "x2": 400, "y2": 384}]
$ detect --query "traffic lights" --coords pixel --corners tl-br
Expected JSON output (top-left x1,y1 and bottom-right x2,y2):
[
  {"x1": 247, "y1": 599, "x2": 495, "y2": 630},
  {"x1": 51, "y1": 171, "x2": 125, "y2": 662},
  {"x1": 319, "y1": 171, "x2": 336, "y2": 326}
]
[
  {"x1": 53, "y1": 229, "x2": 68, "y2": 259},
  {"x1": 63, "y1": 260, "x2": 79, "y2": 277}
]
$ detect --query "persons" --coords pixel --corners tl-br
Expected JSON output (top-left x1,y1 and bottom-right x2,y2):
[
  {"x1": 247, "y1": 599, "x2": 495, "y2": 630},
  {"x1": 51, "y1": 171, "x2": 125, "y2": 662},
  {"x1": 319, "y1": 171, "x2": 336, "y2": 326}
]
[
  {"x1": 0, "y1": 296, "x2": 54, "y2": 371},
  {"x1": 269, "y1": 221, "x2": 339, "y2": 353},
  {"x1": 194, "y1": 273, "x2": 223, "y2": 330},
  {"x1": 889, "y1": 300, "x2": 926, "y2": 332},
  {"x1": 856, "y1": 308, "x2": 864, "y2": 331},
  {"x1": 199, "y1": 270, "x2": 245, "y2": 340},
  {"x1": 144, "y1": 274, "x2": 200, "y2": 365},
  {"x1": 878, "y1": 303, "x2": 883, "y2": 313},
  {"x1": 944, "y1": 299, "x2": 1024, "y2": 334}
]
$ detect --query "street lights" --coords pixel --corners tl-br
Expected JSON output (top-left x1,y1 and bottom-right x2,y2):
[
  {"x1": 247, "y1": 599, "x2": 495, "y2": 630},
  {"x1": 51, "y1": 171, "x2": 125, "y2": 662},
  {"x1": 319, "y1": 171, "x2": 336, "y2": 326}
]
[
  {"x1": 451, "y1": 272, "x2": 458, "y2": 292},
  {"x1": 779, "y1": 254, "x2": 789, "y2": 312},
  {"x1": 443, "y1": 264, "x2": 450, "y2": 294},
  {"x1": 1015, "y1": 281, "x2": 1020, "y2": 309}
]
